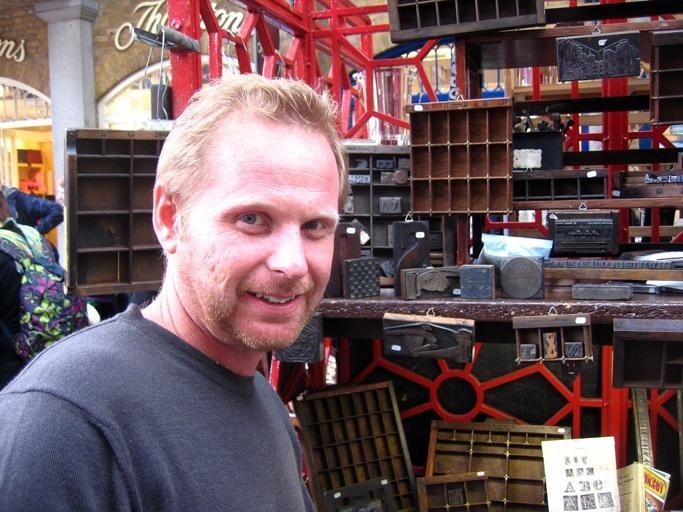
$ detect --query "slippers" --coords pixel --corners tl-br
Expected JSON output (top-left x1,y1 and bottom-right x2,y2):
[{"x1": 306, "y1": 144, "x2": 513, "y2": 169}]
[{"x1": 1, "y1": 234, "x2": 89, "y2": 362}]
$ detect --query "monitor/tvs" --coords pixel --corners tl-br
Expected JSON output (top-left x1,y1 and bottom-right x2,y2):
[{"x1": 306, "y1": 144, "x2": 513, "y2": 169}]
[{"x1": 618, "y1": 461, "x2": 671, "y2": 512}]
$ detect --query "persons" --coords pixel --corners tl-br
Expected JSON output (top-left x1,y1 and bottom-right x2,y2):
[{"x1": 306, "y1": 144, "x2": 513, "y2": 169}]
[
  {"x1": 0, "y1": 190, "x2": 97, "y2": 389},
  {"x1": 0, "y1": 73, "x2": 349, "y2": 512},
  {"x1": 0, "y1": 178, "x2": 66, "y2": 234}
]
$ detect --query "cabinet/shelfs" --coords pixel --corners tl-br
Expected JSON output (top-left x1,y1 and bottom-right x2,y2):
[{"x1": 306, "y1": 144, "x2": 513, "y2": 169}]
[{"x1": 0, "y1": 0, "x2": 683, "y2": 512}]
